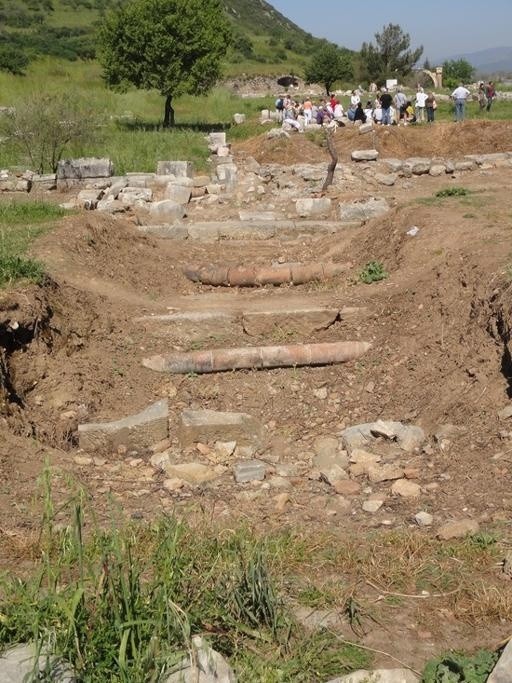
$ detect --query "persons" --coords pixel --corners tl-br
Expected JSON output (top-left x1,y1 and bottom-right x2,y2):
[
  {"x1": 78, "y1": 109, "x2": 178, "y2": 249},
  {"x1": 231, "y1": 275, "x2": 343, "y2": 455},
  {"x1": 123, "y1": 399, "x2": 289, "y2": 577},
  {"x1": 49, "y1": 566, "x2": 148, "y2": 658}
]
[
  {"x1": 275, "y1": 85, "x2": 437, "y2": 127},
  {"x1": 450, "y1": 81, "x2": 471, "y2": 121},
  {"x1": 485, "y1": 79, "x2": 497, "y2": 111},
  {"x1": 475, "y1": 82, "x2": 486, "y2": 111}
]
